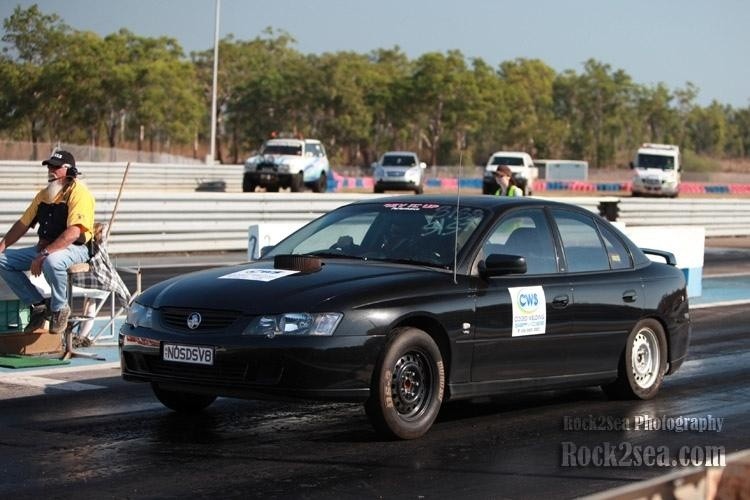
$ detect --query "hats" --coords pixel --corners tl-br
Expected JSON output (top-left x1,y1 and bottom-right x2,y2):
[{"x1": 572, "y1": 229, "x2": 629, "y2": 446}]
[
  {"x1": 491, "y1": 164, "x2": 513, "y2": 178},
  {"x1": 42, "y1": 150, "x2": 76, "y2": 170}
]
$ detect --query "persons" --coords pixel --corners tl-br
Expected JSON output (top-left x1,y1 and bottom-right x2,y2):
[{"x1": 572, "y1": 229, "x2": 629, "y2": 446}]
[
  {"x1": 0, "y1": 151, "x2": 97, "y2": 333},
  {"x1": 492, "y1": 164, "x2": 523, "y2": 197}
]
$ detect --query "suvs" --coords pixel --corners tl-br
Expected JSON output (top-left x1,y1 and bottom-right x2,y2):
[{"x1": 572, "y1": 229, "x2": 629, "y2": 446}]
[
  {"x1": 242, "y1": 136, "x2": 329, "y2": 194},
  {"x1": 371, "y1": 151, "x2": 430, "y2": 194}
]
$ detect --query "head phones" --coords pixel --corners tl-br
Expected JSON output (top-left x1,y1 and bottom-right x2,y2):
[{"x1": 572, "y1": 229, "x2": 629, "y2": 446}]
[{"x1": 66, "y1": 167, "x2": 82, "y2": 177}]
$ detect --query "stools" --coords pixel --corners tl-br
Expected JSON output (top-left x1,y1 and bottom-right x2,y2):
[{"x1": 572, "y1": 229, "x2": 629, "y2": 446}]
[{"x1": 46, "y1": 260, "x2": 108, "y2": 363}]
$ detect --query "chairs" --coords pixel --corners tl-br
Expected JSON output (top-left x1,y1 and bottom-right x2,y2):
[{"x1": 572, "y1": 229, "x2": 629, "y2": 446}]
[{"x1": 502, "y1": 226, "x2": 555, "y2": 275}]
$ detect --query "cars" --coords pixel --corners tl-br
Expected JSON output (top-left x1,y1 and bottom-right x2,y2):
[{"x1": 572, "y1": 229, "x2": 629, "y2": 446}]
[{"x1": 118, "y1": 191, "x2": 691, "y2": 441}]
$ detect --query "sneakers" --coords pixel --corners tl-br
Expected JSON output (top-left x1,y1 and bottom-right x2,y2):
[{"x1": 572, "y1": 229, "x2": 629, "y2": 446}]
[
  {"x1": 23, "y1": 298, "x2": 49, "y2": 335},
  {"x1": 49, "y1": 304, "x2": 72, "y2": 335}
]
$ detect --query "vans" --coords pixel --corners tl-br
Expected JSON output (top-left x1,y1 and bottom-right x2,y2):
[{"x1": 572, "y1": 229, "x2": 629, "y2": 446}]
[
  {"x1": 628, "y1": 141, "x2": 684, "y2": 197},
  {"x1": 480, "y1": 151, "x2": 543, "y2": 189}
]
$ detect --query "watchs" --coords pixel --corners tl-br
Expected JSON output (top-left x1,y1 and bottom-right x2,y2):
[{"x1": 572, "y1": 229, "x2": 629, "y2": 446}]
[{"x1": 41, "y1": 248, "x2": 49, "y2": 256}]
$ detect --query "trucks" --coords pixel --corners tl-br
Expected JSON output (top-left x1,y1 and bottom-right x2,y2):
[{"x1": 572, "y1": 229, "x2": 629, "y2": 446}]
[{"x1": 532, "y1": 159, "x2": 590, "y2": 182}]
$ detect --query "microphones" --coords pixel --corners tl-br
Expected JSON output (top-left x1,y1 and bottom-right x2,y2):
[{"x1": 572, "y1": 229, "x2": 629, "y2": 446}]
[{"x1": 48, "y1": 175, "x2": 75, "y2": 182}]
